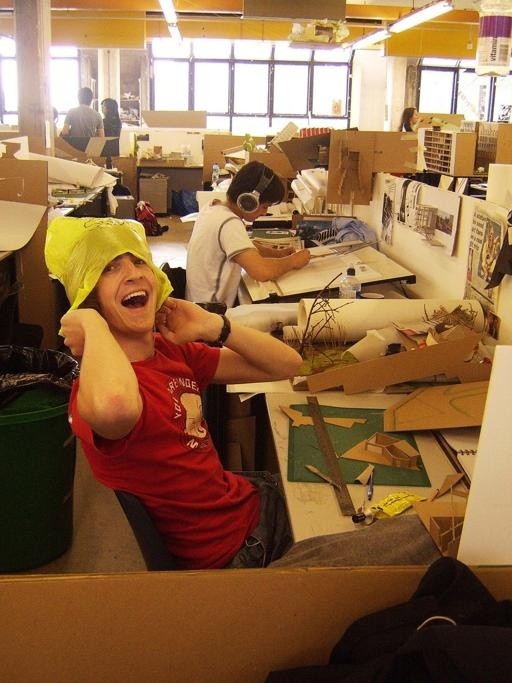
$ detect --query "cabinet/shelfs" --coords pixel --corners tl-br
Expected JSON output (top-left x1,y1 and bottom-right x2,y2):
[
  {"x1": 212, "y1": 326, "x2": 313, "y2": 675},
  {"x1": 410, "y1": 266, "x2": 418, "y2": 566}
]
[{"x1": 138, "y1": 176, "x2": 168, "y2": 214}]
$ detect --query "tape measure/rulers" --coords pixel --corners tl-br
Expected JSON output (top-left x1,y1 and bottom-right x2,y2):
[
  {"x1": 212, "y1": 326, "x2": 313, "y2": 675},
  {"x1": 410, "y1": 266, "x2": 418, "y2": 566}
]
[{"x1": 306, "y1": 396, "x2": 357, "y2": 516}]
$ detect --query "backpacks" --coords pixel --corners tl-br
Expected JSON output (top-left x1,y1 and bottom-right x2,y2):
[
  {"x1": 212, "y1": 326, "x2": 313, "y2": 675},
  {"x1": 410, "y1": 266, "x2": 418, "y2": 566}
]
[{"x1": 135, "y1": 200, "x2": 168, "y2": 236}]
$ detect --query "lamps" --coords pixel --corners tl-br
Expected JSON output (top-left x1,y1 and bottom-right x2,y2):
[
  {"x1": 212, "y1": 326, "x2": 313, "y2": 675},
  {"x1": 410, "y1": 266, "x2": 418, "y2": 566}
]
[
  {"x1": 343, "y1": 0, "x2": 391, "y2": 51},
  {"x1": 389, "y1": 0, "x2": 454, "y2": 34}
]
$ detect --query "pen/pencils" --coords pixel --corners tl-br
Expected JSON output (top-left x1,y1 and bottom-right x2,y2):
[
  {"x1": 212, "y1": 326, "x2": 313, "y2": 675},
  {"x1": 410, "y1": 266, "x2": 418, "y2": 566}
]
[
  {"x1": 301, "y1": 238, "x2": 305, "y2": 249},
  {"x1": 305, "y1": 465, "x2": 341, "y2": 490},
  {"x1": 367, "y1": 469, "x2": 374, "y2": 501}
]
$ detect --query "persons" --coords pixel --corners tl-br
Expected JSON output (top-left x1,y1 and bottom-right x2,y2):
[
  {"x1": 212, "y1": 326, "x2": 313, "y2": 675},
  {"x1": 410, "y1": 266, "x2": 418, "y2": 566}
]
[
  {"x1": 62, "y1": 84, "x2": 106, "y2": 153},
  {"x1": 52, "y1": 102, "x2": 60, "y2": 140},
  {"x1": 397, "y1": 106, "x2": 418, "y2": 132},
  {"x1": 99, "y1": 94, "x2": 122, "y2": 158},
  {"x1": 180, "y1": 156, "x2": 313, "y2": 306},
  {"x1": 40, "y1": 213, "x2": 307, "y2": 567}
]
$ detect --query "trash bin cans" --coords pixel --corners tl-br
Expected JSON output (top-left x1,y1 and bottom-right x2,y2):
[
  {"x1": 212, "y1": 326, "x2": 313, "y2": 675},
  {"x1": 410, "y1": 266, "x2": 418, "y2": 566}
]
[{"x1": 0, "y1": 346, "x2": 78, "y2": 571}]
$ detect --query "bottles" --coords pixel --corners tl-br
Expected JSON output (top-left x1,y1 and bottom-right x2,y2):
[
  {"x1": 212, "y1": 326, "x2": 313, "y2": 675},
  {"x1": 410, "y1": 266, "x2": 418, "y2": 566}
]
[
  {"x1": 338, "y1": 267, "x2": 362, "y2": 301},
  {"x1": 211, "y1": 162, "x2": 220, "y2": 181},
  {"x1": 105, "y1": 155, "x2": 113, "y2": 170}
]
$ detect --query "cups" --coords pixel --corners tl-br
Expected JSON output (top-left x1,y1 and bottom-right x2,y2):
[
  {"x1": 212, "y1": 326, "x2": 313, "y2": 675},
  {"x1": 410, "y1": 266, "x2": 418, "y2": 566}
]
[{"x1": 360, "y1": 291, "x2": 384, "y2": 299}]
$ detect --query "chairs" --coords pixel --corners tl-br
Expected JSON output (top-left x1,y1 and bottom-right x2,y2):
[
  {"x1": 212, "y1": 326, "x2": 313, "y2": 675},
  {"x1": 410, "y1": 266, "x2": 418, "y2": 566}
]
[
  {"x1": 159, "y1": 262, "x2": 171, "y2": 273},
  {"x1": 114, "y1": 488, "x2": 178, "y2": 570}
]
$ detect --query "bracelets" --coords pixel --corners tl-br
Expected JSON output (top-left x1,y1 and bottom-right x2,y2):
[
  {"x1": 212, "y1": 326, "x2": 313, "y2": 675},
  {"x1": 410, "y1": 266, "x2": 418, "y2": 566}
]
[{"x1": 210, "y1": 310, "x2": 232, "y2": 349}]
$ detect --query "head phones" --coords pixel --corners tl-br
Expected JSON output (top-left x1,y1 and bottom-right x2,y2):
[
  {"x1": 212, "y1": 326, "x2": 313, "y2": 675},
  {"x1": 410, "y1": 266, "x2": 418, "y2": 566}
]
[{"x1": 237, "y1": 166, "x2": 275, "y2": 213}]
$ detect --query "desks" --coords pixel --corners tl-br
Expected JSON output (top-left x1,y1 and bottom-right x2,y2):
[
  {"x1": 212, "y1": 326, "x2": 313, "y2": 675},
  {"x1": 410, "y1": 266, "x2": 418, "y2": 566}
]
[{"x1": 264, "y1": 391, "x2": 469, "y2": 544}]
